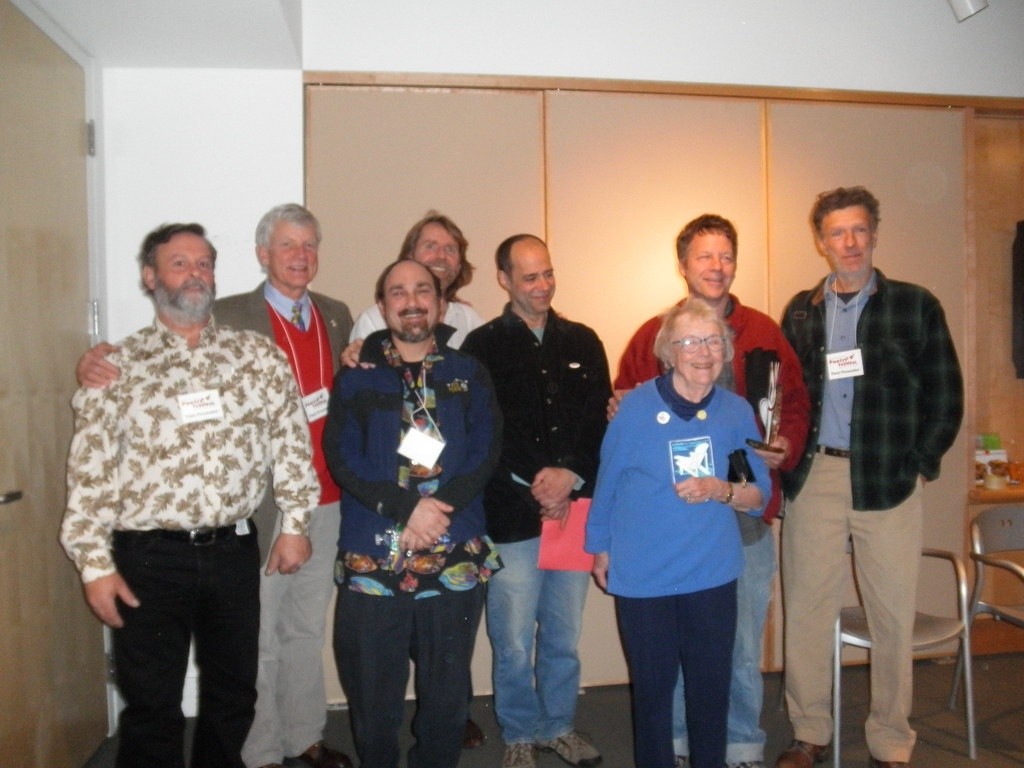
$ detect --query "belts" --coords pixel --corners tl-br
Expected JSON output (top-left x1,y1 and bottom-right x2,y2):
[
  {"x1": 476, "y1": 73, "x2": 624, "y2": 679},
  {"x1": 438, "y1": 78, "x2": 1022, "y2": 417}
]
[
  {"x1": 114, "y1": 524, "x2": 236, "y2": 548},
  {"x1": 815, "y1": 444, "x2": 851, "y2": 459}
]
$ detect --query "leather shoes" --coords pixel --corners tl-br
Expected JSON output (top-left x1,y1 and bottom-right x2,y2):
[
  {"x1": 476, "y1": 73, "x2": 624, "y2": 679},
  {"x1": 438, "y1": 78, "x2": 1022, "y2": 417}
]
[
  {"x1": 297, "y1": 745, "x2": 354, "y2": 768},
  {"x1": 776, "y1": 741, "x2": 830, "y2": 768},
  {"x1": 872, "y1": 757, "x2": 913, "y2": 768},
  {"x1": 464, "y1": 718, "x2": 484, "y2": 749}
]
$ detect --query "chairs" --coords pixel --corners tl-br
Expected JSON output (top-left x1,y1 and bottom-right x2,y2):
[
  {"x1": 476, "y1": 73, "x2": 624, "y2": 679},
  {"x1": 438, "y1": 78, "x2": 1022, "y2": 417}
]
[
  {"x1": 778, "y1": 543, "x2": 978, "y2": 768},
  {"x1": 945, "y1": 503, "x2": 1024, "y2": 712}
]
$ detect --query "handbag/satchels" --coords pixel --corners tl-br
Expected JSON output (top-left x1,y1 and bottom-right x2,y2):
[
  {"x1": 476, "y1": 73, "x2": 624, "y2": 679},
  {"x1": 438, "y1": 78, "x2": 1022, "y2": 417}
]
[{"x1": 727, "y1": 449, "x2": 756, "y2": 482}]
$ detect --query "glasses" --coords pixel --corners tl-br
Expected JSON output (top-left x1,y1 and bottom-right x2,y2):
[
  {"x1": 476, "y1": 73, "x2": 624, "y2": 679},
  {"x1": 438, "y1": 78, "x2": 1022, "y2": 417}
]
[{"x1": 672, "y1": 334, "x2": 727, "y2": 352}]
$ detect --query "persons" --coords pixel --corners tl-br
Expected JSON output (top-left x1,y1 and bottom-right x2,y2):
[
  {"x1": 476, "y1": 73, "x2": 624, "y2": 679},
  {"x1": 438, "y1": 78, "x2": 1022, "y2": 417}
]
[
  {"x1": 60, "y1": 224, "x2": 320, "y2": 768},
  {"x1": 75, "y1": 203, "x2": 355, "y2": 768},
  {"x1": 779, "y1": 185, "x2": 966, "y2": 768},
  {"x1": 586, "y1": 297, "x2": 772, "y2": 768},
  {"x1": 606, "y1": 214, "x2": 811, "y2": 768},
  {"x1": 459, "y1": 234, "x2": 613, "y2": 768},
  {"x1": 323, "y1": 259, "x2": 505, "y2": 768},
  {"x1": 340, "y1": 212, "x2": 487, "y2": 749}
]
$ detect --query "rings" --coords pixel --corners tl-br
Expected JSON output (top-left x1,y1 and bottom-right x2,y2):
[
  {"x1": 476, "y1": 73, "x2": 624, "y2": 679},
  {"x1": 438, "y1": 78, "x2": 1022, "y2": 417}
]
[{"x1": 687, "y1": 493, "x2": 690, "y2": 500}]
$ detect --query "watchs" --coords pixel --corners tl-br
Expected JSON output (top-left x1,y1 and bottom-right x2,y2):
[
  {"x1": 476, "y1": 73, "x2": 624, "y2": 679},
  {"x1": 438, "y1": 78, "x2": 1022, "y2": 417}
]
[{"x1": 720, "y1": 481, "x2": 734, "y2": 505}]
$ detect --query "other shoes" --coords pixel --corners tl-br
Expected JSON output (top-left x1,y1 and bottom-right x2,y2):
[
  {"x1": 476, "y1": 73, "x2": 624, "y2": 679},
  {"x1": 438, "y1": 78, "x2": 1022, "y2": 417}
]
[
  {"x1": 729, "y1": 762, "x2": 767, "y2": 768},
  {"x1": 674, "y1": 756, "x2": 691, "y2": 768}
]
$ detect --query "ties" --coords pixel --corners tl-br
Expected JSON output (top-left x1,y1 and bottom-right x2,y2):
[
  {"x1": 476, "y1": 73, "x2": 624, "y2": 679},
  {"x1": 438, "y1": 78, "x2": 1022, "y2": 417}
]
[{"x1": 291, "y1": 301, "x2": 306, "y2": 332}]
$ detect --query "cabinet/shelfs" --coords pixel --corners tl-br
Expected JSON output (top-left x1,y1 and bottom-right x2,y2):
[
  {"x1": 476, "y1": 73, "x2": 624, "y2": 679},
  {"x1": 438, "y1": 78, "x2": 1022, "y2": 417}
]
[{"x1": 966, "y1": 482, "x2": 1024, "y2": 656}]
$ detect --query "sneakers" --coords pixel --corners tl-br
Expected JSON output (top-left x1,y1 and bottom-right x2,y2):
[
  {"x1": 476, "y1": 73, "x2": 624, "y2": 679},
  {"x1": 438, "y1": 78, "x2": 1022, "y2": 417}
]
[
  {"x1": 537, "y1": 729, "x2": 603, "y2": 768},
  {"x1": 503, "y1": 744, "x2": 538, "y2": 768}
]
